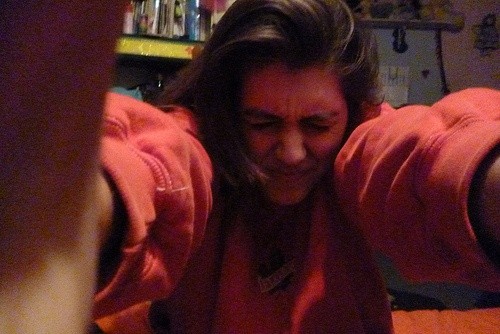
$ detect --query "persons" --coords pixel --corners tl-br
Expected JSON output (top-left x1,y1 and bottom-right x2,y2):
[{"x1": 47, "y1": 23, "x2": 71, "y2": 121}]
[{"x1": 0, "y1": 0, "x2": 500, "y2": 334}]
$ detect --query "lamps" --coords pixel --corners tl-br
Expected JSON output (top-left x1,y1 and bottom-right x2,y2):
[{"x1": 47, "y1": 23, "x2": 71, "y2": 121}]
[
  {"x1": 470, "y1": 13, "x2": 500, "y2": 55},
  {"x1": 392, "y1": 27, "x2": 408, "y2": 53}
]
